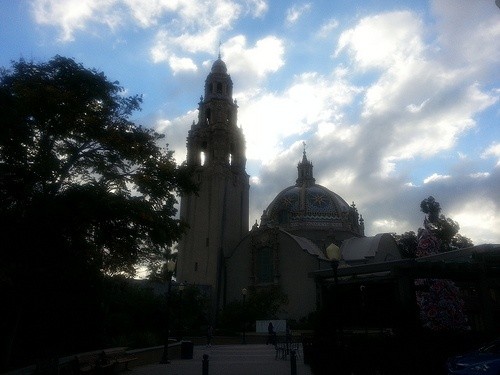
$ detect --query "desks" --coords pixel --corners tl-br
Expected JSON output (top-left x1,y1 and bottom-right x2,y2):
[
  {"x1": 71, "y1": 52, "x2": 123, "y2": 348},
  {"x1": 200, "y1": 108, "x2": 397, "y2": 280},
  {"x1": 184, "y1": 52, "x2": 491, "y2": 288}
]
[{"x1": 111, "y1": 357, "x2": 139, "y2": 371}]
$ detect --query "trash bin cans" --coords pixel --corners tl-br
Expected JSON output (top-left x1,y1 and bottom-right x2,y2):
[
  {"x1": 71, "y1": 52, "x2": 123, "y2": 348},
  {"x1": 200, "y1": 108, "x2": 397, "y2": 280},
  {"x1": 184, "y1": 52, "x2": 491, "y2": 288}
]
[
  {"x1": 302, "y1": 338, "x2": 314, "y2": 363},
  {"x1": 182, "y1": 342, "x2": 193, "y2": 359}
]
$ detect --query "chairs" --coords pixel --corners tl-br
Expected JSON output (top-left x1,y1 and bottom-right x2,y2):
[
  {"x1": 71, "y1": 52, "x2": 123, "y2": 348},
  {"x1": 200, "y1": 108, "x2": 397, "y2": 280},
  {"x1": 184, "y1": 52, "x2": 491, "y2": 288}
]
[{"x1": 274, "y1": 342, "x2": 302, "y2": 360}]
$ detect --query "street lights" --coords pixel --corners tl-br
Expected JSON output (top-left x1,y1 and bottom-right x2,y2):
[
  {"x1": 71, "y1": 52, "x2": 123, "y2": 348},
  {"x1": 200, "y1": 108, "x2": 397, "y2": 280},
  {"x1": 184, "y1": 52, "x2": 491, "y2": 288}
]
[
  {"x1": 178, "y1": 282, "x2": 184, "y2": 340},
  {"x1": 241, "y1": 287, "x2": 247, "y2": 345},
  {"x1": 326, "y1": 243, "x2": 344, "y2": 375}
]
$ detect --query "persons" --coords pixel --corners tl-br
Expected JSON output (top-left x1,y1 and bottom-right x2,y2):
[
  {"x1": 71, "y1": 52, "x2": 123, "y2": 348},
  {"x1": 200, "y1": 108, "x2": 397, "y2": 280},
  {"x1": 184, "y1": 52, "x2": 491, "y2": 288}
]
[
  {"x1": 266, "y1": 322, "x2": 276, "y2": 345},
  {"x1": 205, "y1": 325, "x2": 212, "y2": 347}
]
